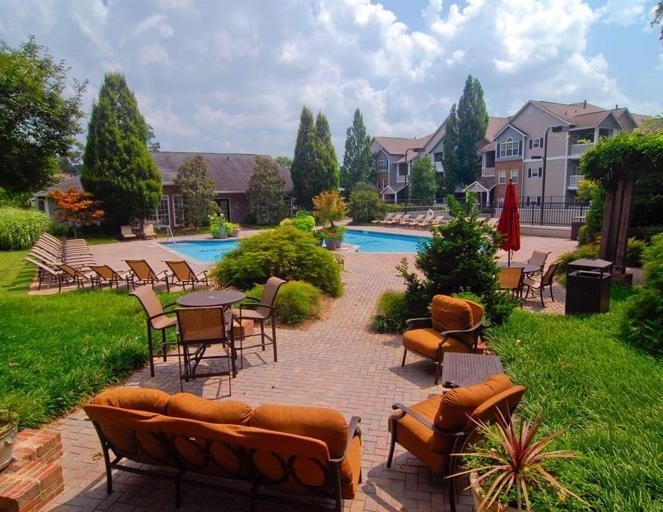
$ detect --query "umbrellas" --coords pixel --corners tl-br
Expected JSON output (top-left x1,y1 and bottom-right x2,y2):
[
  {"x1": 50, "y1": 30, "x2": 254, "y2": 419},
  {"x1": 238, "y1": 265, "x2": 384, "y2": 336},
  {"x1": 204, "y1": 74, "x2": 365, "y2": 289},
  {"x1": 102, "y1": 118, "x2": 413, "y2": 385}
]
[{"x1": 491, "y1": 183, "x2": 521, "y2": 268}]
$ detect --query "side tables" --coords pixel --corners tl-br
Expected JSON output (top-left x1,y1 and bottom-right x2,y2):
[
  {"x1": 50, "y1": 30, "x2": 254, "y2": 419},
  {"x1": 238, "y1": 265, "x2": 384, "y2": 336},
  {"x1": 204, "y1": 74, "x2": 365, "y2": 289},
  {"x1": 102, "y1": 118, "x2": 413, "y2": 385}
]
[{"x1": 441, "y1": 351, "x2": 505, "y2": 395}]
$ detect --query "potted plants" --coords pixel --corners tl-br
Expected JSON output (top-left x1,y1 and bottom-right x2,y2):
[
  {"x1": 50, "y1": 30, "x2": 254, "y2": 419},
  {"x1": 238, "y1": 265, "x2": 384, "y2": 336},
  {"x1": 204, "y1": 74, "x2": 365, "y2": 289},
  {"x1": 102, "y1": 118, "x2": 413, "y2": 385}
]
[
  {"x1": 206, "y1": 212, "x2": 241, "y2": 239},
  {"x1": 443, "y1": 399, "x2": 594, "y2": 512},
  {"x1": 0, "y1": 396, "x2": 19, "y2": 472}
]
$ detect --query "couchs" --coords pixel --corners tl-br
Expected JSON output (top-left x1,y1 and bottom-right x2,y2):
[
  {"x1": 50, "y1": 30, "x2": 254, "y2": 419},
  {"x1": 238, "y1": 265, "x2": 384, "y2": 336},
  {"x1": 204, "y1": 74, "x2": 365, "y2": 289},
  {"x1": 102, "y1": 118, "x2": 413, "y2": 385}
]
[{"x1": 78, "y1": 385, "x2": 362, "y2": 511}]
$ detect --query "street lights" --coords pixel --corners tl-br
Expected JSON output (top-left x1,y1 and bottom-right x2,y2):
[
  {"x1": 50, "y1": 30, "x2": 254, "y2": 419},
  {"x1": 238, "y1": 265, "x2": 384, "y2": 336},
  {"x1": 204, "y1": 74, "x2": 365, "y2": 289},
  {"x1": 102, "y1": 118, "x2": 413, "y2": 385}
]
[
  {"x1": 401, "y1": 147, "x2": 426, "y2": 215},
  {"x1": 538, "y1": 125, "x2": 569, "y2": 229}
]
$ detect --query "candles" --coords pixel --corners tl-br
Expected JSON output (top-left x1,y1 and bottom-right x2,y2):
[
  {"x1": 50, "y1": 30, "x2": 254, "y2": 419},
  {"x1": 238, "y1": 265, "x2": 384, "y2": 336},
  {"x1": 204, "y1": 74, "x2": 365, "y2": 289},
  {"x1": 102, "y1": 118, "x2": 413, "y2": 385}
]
[{"x1": 288, "y1": 189, "x2": 345, "y2": 251}]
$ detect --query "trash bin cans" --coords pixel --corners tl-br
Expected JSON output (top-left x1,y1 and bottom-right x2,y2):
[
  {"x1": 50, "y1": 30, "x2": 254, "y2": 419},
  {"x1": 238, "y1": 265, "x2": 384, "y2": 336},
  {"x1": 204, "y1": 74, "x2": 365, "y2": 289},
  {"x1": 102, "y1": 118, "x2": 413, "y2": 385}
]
[
  {"x1": 571, "y1": 218, "x2": 586, "y2": 240},
  {"x1": 565, "y1": 258, "x2": 613, "y2": 315}
]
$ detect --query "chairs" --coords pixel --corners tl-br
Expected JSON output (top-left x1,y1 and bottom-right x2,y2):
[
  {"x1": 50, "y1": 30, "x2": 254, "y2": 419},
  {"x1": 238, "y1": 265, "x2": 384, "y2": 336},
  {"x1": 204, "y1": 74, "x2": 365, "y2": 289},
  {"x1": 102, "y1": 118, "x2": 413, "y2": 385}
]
[
  {"x1": 128, "y1": 276, "x2": 288, "y2": 396},
  {"x1": 386, "y1": 376, "x2": 526, "y2": 512},
  {"x1": 402, "y1": 293, "x2": 485, "y2": 385},
  {"x1": 369, "y1": 211, "x2": 500, "y2": 235},
  {"x1": 494, "y1": 248, "x2": 560, "y2": 311},
  {"x1": 53, "y1": 257, "x2": 209, "y2": 294},
  {"x1": 120, "y1": 223, "x2": 157, "y2": 240},
  {"x1": 22, "y1": 231, "x2": 97, "y2": 290}
]
